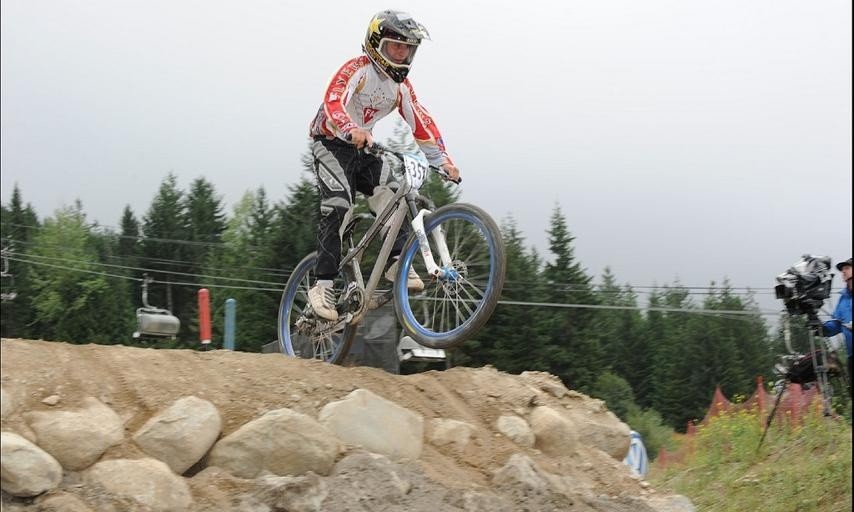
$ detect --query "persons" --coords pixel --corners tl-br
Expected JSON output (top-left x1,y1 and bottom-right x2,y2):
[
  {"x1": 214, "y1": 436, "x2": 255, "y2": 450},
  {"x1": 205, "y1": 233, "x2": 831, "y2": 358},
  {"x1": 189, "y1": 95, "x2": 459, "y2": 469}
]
[
  {"x1": 814, "y1": 258, "x2": 853, "y2": 401},
  {"x1": 309, "y1": 10, "x2": 462, "y2": 321}
]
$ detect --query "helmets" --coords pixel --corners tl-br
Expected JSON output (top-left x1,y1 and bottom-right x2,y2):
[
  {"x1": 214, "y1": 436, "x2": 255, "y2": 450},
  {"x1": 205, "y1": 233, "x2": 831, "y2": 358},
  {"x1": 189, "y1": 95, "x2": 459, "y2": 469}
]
[{"x1": 362, "y1": 10, "x2": 430, "y2": 83}]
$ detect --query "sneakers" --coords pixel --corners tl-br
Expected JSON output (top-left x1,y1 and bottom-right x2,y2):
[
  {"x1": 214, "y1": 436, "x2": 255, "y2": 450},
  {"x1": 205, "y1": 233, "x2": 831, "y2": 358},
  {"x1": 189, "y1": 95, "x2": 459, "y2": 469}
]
[
  {"x1": 385, "y1": 256, "x2": 425, "y2": 290},
  {"x1": 307, "y1": 283, "x2": 339, "y2": 320}
]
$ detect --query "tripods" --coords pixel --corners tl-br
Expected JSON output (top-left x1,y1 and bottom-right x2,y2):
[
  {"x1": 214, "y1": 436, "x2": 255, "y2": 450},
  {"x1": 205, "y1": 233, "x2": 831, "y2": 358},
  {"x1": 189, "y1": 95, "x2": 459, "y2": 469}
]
[{"x1": 755, "y1": 311, "x2": 854, "y2": 455}]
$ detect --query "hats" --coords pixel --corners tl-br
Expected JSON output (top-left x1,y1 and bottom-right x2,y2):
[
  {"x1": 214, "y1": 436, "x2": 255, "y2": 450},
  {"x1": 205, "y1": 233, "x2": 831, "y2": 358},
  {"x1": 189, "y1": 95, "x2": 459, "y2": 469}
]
[{"x1": 837, "y1": 258, "x2": 852, "y2": 271}]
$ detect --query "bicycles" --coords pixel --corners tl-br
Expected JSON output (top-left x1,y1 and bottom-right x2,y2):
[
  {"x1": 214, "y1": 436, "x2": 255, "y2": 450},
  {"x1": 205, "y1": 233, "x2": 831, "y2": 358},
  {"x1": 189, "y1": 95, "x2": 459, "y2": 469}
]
[{"x1": 276, "y1": 131, "x2": 507, "y2": 366}]
